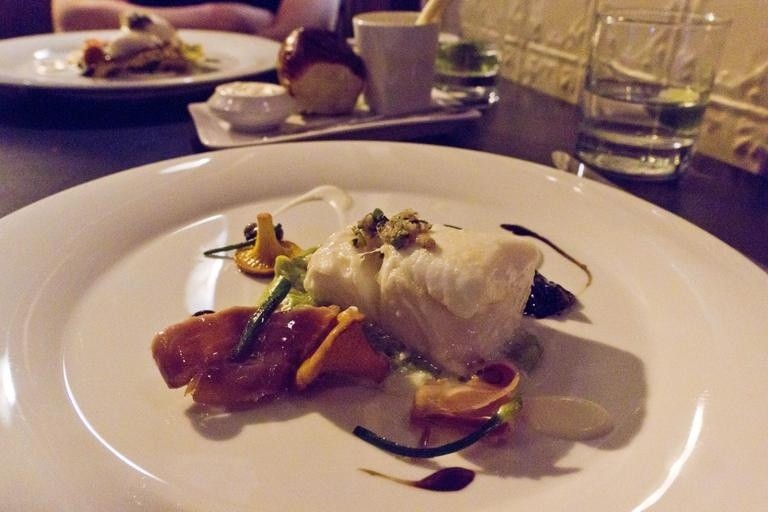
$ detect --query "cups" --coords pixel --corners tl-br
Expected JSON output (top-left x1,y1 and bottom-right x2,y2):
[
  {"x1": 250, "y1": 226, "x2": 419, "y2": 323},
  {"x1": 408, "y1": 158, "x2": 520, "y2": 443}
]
[
  {"x1": 575, "y1": 14, "x2": 732, "y2": 184},
  {"x1": 350, "y1": 11, "x2": 444, "y2": 116}
]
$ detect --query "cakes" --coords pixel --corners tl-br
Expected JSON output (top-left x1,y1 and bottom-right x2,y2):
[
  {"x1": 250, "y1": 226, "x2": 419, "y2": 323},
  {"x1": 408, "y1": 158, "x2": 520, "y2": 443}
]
[{"x1": 276, "y1": 25, "x2": 367, "y2": 118}]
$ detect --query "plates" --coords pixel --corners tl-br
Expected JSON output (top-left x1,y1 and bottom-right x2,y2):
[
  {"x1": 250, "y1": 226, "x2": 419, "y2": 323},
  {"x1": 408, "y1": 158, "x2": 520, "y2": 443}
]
[
  {"x1": 346, "y1": 30, "x2": 501, "y2": 77},
  {"x1": 1, "y1": 138, "x2": 768, "y2": 510},
  {"x1": 189, "y1": 84, "x2": 482, "y2": 148},
  {"x1": 0, "y1": 31, "x2": 283, "y2": 94}
]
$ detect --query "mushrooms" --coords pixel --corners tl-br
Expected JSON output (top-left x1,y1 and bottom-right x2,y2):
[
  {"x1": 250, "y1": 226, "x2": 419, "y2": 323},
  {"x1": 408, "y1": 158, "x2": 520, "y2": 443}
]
[
  {"x1": 234, "y1": 211, "x2": 303, "y2": 277},
  {"x1": 294, "y1": 305, "x2": 394, "y2": 389}
]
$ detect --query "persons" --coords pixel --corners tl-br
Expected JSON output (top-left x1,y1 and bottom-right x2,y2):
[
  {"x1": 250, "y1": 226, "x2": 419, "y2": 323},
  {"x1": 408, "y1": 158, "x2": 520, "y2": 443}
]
[{"x1": 49, "y1": 2, "x2": 340, "y2": 42}]
